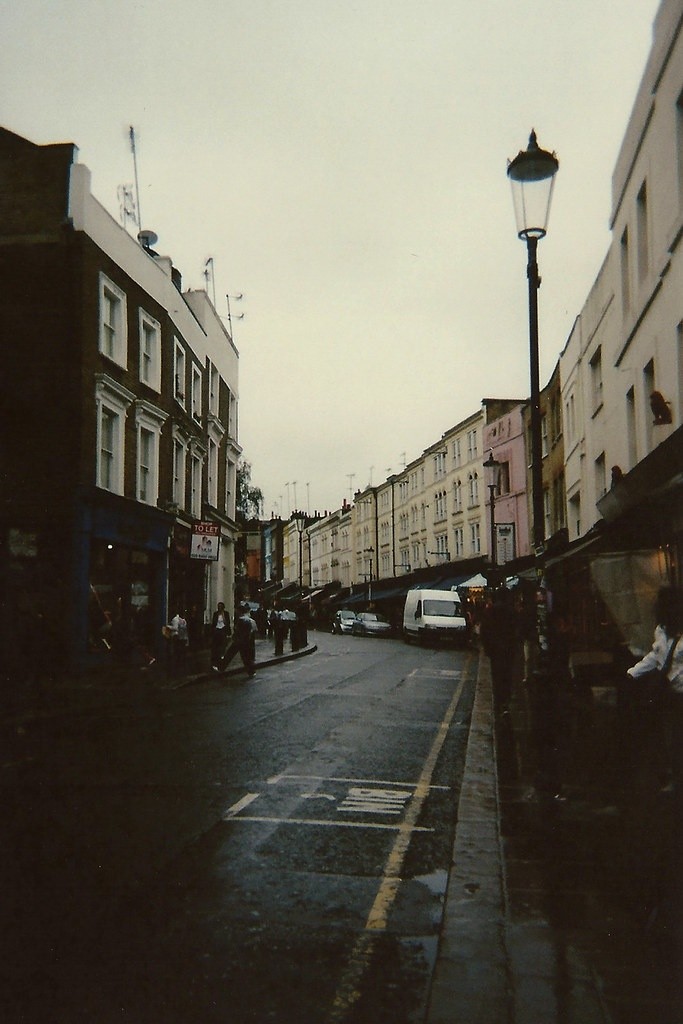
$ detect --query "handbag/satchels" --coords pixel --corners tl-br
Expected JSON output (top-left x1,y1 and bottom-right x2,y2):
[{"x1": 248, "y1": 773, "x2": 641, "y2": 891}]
[{"x1": 632, "y1": 669, "x2": 672, "y2": 712}]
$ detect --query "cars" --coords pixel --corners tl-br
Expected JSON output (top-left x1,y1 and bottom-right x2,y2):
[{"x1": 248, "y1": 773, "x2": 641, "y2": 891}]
[
  {"x1": 350, "y1": 612, "x2": 394, "y2": 639},
  {"x1": 331, "y1": 610, "x2": 356, "y2": 635}
]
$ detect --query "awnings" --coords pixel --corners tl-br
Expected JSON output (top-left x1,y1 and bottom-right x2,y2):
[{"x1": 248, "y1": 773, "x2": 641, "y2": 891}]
[{"x1": 258, "y1": 523, "x2": 609, "y2": 610}]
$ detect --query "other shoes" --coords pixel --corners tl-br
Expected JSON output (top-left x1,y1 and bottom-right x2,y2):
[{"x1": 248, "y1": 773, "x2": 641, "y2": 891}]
[
  {"x1": 249, "y1": 672, "x2": 256, "y2": 678},
  {"x1": 213, "y1": 666, "x2": 223, "y2": 673}
]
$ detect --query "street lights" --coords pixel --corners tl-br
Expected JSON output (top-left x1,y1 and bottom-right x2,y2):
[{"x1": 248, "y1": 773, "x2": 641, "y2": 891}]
[
  {"x1": 367, "y1": 546, "x2": 375, "y2": 603},
  {"x1": 295, "y1": 511, "x2": 307, "y2": 588},
  {"x1": 505, "y1": 131, "x2": 562, "y2": 564}
]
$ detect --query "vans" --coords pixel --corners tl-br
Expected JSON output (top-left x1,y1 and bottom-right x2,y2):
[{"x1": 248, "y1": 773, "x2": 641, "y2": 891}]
[{"x1": 402, "y1": 589, "x2": 467, "y2": 645}]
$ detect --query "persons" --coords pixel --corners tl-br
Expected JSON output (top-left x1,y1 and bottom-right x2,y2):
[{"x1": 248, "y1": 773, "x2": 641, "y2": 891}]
[
  {"x1": 627, "y1": 588, "x2": 683, "y2": 791},
  {"x1": 210, "y1": 603, "x2": 258, "y2": 679},
  {"x1": 244, "y1": 603, "x2": 296, "y2": 640},
  {"x1": 212, "y1": 602, "x2": 231, "y2": 658}
]
